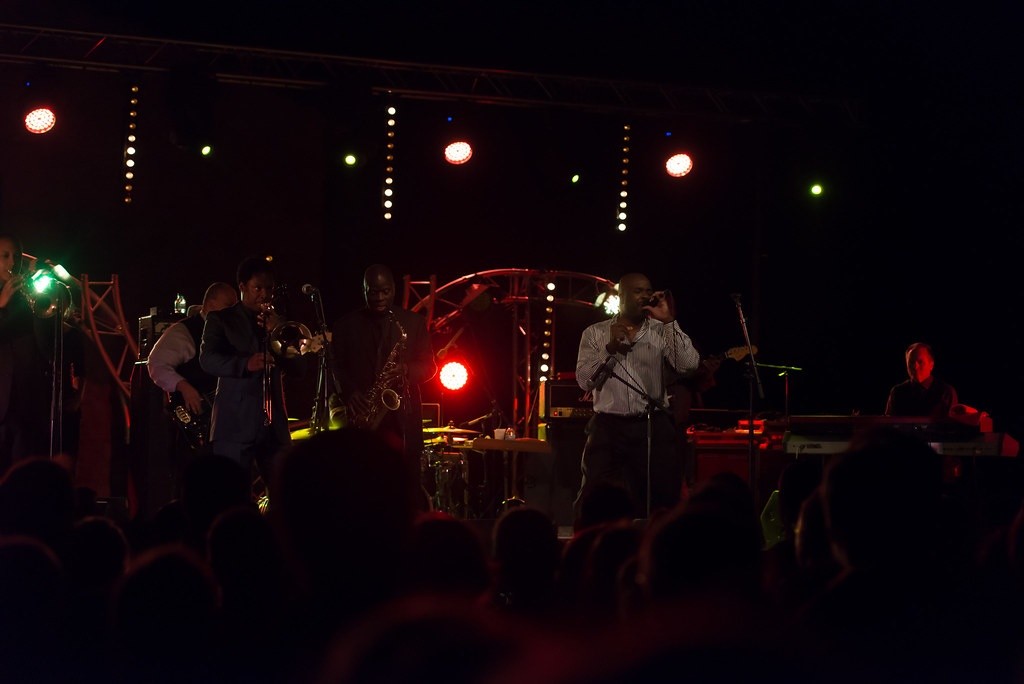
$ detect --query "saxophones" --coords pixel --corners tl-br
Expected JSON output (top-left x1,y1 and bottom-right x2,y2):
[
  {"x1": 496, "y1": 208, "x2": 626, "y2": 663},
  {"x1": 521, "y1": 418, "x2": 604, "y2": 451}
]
[{"x1": 356, "y1": 309, "x2": 407, "y2": 433}]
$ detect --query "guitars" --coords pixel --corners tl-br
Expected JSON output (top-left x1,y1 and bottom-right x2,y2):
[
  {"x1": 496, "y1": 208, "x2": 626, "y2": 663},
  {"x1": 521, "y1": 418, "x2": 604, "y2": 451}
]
[
  {"x1": 666, "y1": 343, "x2": 757, "y2": 385},
  {"x1": 168, "y1": 330, "x2": 332, "y2": 451}
]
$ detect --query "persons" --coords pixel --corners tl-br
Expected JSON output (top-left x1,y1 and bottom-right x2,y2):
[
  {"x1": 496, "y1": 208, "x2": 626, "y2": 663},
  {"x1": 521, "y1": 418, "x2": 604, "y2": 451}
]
[
  {"x1": 564, "y1": 274, "x2": 700, "y2": 537},
  {"x1": 196, "y1": 259, "x2": 291, "y2": 532},
  {"x1": 0, "y1": 231, "x2": 51, "y2": 477},
  {"x1": 144, "y1": 281, "x2": 238, "y2": 482},
  {"x1": 881, "y1": 344, "x2": 978, "y2": 431},
  {"x1": 0, "y1": 427, "x2": 1024, "y2": 684},
  {"x1": 329, "y1": 262, "x2": 438, "y2": 519},
  {"x1": 690, "y1": 351, "x2": 735, "y2": 424}
]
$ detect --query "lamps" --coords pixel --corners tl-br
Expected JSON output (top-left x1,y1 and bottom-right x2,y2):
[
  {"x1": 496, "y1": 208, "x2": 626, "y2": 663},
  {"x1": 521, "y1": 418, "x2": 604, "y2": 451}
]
[{"x1": 9, "y1": 61, "x2": 698, "y2": 178}]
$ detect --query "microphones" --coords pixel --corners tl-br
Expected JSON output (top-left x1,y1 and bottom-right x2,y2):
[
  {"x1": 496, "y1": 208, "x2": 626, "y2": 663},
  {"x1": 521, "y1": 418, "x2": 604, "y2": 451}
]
[
  {"x1": 301, "y1": 284, "x2": 319, "y2": 295},
  {"x1": 468, "y1": 413, "x2": 494, "y2": 426},
  {"x1": 644, "y1": 290, "x2": 668, "y2": 308},
  {"x1": 586, "y1": 355, "x2": 611, "y2": 387}
]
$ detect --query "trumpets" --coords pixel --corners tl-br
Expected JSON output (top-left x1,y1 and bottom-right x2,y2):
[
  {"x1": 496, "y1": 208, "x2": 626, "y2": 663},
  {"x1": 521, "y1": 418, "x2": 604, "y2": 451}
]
[
  {"x1": 261, "y1": 308, "x2": 312, "y2": 360},
  {"x1": 9, "y1": 270, "x2": 55, "y2": 318}
]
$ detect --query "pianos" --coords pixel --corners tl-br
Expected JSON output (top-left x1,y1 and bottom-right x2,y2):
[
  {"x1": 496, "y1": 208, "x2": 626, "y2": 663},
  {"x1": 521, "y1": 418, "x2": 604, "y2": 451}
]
[{"x1": 757, "y1": 415, "x2": 997, "y2": 454}]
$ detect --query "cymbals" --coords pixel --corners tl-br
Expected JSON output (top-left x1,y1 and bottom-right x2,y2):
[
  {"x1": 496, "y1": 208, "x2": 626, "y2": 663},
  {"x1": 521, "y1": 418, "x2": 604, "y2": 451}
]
[{"x1": 422, "y1": 425, "x2": 481, "y2": 435}]
